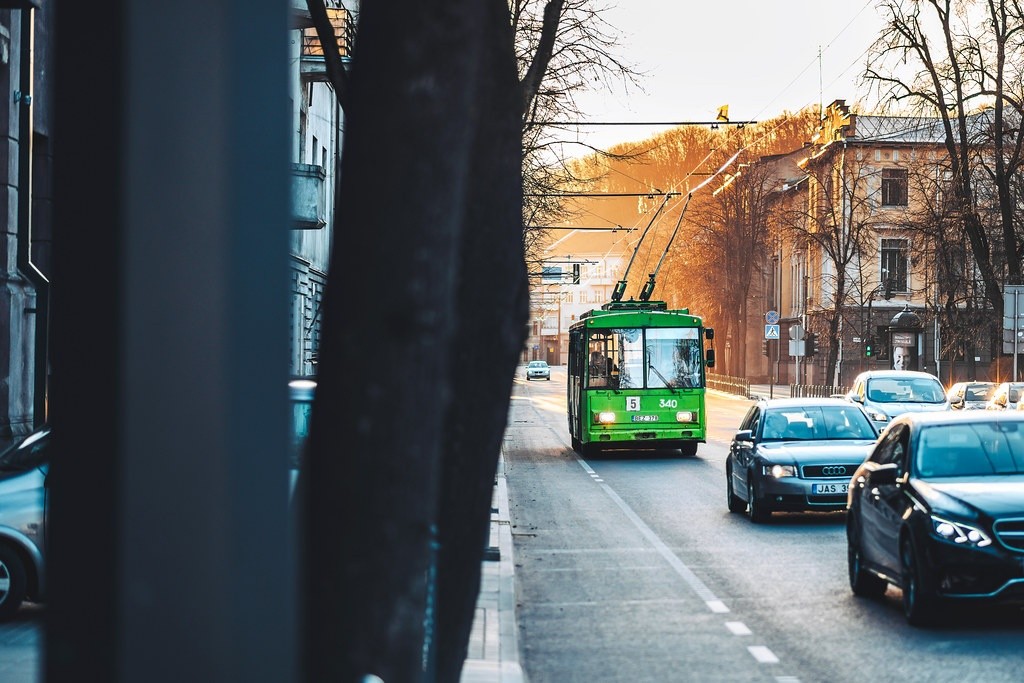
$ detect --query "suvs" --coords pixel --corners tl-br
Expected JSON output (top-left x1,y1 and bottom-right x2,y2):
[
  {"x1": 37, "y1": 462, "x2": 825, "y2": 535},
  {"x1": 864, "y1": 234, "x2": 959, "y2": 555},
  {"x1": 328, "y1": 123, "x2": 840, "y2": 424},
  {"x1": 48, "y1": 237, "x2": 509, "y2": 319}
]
[{"x1": 726, "y1": 394, "x2": 881, "y2": 525}]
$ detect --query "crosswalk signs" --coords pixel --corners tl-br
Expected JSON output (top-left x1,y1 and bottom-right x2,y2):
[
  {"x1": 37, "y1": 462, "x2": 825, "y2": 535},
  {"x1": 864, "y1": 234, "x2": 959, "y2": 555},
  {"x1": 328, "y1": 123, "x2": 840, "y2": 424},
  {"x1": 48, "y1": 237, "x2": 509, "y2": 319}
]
[{"x1": 765, "y1": 325, "x2": 779, "y2": 340}]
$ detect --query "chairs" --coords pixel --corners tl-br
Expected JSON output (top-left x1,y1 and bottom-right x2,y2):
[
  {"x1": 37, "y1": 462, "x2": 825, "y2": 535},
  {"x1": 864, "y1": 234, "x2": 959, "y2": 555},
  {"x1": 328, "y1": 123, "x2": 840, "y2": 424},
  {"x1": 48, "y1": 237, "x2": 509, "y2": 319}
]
[
  {"x1": 967, "y1": 391, "x2": 974, "y2": 396},
  {"x1": 871, "y1": 390, "x2": 883, "y2": 401}
]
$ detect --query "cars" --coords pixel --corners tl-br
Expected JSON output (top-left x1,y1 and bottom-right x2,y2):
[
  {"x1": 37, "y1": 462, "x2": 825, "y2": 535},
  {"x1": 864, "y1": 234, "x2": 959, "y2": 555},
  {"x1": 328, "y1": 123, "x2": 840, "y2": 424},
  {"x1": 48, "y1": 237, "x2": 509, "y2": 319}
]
[
  {"x1": 843, "y1": 371, "x2": 1024, "y2": 468},
  {"x1": 845, "y1": 411, "x2": 1024, "y2": 628},
  {"x1": 525, "y1": 361, "x2": 551, "y2": 380}
]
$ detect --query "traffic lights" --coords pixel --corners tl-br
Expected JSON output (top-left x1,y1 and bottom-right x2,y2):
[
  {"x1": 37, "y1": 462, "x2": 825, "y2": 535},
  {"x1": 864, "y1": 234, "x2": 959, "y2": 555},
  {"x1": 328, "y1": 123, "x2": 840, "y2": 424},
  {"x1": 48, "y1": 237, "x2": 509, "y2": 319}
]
[
  {"x1": 865, "y1": 340, "x2": 874, "y2": 357},
  {"x1": 885, "y1": 279, "x2": 896, "y2": 300},
  {"x1": 805, "y1": 333, "x2": 819, "y2": 357}
]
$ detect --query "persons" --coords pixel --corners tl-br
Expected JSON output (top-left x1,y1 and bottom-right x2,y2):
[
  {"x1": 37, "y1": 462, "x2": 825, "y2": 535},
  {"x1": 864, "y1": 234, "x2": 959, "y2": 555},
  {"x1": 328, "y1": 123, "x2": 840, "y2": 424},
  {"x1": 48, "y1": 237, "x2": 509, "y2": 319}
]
[
  {"x1": 766, "y1": 416, "x2": 795, "y2": 438},
  {"x1": 892, "y1": 346, "x2": 910, "y2": 370},
  {"x1": 661, "y1": 347, "x2": 687, "y2": 376},
  {"x1": 589, "y1": 352, "x2": 606, "y2": 376},
  {"x1": 607, "y1": 358, "x2": 618, "y2": 371},
  {"x1": 817, "y1": 414, "x2": 844, "y2": 434}
]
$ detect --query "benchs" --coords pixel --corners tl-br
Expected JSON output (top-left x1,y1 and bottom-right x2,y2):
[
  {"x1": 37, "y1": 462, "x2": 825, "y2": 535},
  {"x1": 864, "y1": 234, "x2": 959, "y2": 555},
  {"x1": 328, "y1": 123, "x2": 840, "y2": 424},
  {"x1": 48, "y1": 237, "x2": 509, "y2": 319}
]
[{"x1": 789, "y1": 421, "x2": 813, "y2": 435}]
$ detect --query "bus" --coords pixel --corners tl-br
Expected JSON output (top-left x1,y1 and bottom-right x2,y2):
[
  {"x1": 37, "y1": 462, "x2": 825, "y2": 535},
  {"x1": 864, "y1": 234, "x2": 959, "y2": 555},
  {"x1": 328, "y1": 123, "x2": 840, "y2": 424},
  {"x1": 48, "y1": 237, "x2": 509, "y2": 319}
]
[{"x1": 567, "y1": 193, "x2": 715, "y2": 459}]
